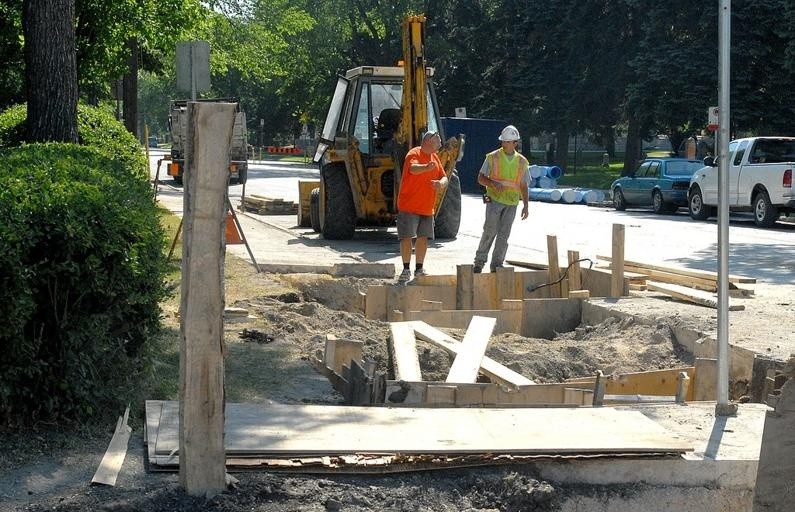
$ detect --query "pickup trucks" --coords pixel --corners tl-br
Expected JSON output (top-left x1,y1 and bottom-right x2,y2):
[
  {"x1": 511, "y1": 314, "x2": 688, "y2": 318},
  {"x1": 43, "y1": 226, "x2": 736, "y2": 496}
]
[{"x1": 685, "y1": 135, "x2": 795, "y2": 230}]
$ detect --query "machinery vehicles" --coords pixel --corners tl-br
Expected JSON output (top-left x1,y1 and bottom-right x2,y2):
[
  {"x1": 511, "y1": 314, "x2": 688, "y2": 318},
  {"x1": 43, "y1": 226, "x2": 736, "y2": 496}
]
[{"x1": 294, "y1": 7, "x2": 465, "y2": 248}]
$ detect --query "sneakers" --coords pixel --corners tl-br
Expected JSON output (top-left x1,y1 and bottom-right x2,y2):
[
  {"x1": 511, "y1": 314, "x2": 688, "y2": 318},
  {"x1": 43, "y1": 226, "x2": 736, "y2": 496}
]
[
  {"x1": 473, "y1": 267, "x2": 481, "y2": 273},
  {"x1": 414, "y1": 270, "x2": 428, "y2": 278},
  {"x1": 398, "y1": 269, "x2": 411, "y2": 281}
]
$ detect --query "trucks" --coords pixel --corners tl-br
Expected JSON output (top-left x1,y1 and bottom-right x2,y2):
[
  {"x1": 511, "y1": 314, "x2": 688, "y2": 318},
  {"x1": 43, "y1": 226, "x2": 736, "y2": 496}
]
[{"x1": 161, "y1": 97, "x2": 252, "y2": 189}]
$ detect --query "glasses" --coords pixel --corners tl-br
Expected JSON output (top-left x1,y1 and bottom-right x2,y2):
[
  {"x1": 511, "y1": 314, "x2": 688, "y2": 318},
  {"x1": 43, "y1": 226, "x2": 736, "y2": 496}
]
[{"x1": 430, "y1": 132, "x2": 440, "y2": 139}]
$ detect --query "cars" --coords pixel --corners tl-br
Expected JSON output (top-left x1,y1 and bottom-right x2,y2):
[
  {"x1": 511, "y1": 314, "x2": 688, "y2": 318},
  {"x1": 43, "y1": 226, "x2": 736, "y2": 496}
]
[
  {"x1": 678, "y1": 136, "x2": 712, "y2": 156},
  {"x1": 609, "y1": 154, "x2": 712, "y2": 215}
]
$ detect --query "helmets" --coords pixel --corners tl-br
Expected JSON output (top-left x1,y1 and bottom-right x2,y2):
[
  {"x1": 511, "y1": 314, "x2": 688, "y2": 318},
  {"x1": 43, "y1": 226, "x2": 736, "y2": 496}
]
[{"x1": 498, "y1": 125, "x2": 521, "y2": 142}]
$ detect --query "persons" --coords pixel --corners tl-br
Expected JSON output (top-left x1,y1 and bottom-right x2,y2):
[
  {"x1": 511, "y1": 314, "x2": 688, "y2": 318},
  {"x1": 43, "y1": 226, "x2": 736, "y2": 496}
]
[
  {"x1": 395, "y1": 130, "x2": 449, "y2": 282},
  {"x1": 470, "y1": 124, "x2": 530, "y2": 275}
]
